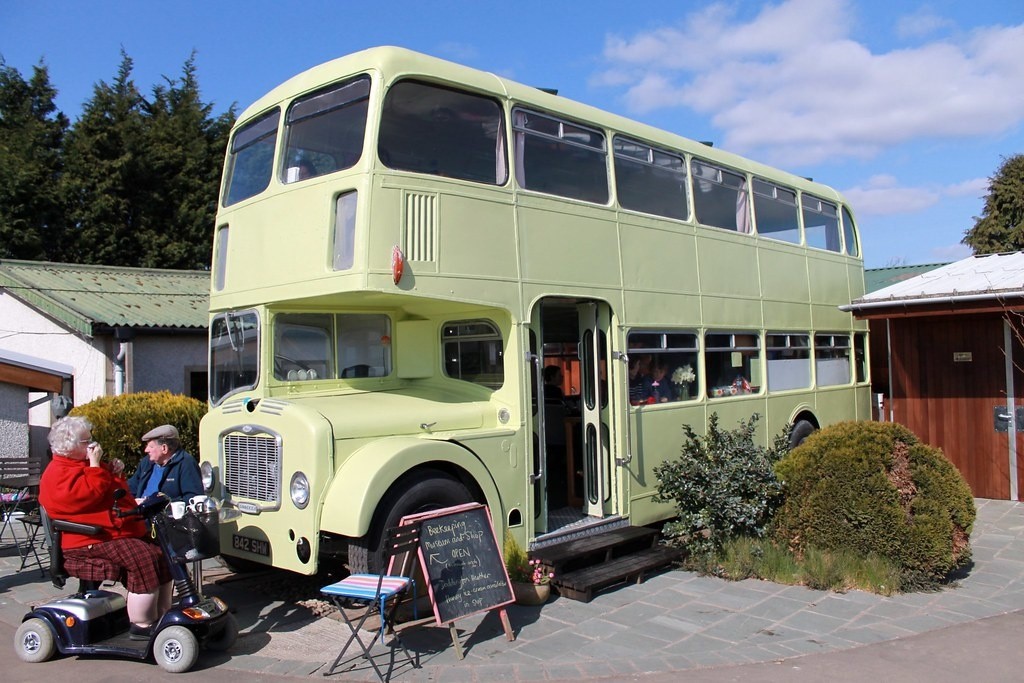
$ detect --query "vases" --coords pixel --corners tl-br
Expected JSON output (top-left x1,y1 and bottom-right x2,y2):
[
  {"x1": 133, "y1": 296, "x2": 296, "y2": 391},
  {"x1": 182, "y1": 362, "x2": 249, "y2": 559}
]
[
  {"x1": 511, "y1": 578, "x2": 551, "y2": 606},
  {"x1": 677, "y1": 381, "x2": 690, "y2": 401}
]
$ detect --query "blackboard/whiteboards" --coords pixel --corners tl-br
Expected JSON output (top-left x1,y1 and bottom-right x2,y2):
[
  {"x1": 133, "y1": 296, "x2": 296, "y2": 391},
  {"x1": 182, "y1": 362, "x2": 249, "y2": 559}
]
[{"x1": 410, "y1": 504, "x2": 518, "y2": 626}]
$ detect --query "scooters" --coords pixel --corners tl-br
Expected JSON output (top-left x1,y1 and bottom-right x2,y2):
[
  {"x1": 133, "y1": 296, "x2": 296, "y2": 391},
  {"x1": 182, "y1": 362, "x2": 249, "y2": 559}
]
[{"x1": 14, "y1": 488, "x2": 239, "y2": 673}]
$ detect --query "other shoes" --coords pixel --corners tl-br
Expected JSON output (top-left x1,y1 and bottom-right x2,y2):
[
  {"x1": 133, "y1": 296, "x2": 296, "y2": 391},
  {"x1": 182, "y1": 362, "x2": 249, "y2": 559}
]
[{"x1": 129, "y1": 619, "x2": 159, "y2": 640}]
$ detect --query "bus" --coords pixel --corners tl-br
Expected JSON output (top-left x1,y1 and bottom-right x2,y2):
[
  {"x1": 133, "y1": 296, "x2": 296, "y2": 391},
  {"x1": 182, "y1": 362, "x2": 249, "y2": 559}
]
[{"x1": 198, "y1": 45, "x2": 873, "y2": 623}]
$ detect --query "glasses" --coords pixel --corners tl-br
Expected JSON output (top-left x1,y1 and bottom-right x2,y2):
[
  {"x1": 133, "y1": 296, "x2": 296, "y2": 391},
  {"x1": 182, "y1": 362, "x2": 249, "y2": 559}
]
[{"x1": 79, "y1": 437, "x2": 93, "y2": 443}]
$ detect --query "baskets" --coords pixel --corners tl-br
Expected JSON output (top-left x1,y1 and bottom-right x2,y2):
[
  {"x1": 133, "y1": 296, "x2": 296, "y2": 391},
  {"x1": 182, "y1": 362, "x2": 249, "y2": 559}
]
[{"x1": 165, "y1": 510, "x2": 220, "y2": 563}]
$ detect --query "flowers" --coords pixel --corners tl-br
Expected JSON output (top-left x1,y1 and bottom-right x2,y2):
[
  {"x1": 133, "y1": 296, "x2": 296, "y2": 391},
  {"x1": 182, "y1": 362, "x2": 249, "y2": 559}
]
[
  {"x1": 512, "y1": 559, "x2": 555, "y2": 584},
  {"x1": 670, "y1": 364, "x2": 695, "y2": 385}
]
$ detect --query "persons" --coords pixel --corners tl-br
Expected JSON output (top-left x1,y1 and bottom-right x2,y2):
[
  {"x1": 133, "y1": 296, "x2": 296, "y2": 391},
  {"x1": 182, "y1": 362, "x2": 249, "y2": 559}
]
[
  {"x1": 641, "y1": 360, "x2": 674, "y2": 402},
  {"x1": 627, "y1": 354, "x2": 645, "y2": 400},
  {"x1": 293, "y1": 159, "x2": 316, "y2": 180},
  {"x1": 37, "y1": 415, "x2": 173, "y2": 640},
  {"x1": 125, "y1": 424, "x2": 204, "y2": 533},
  {"x1": 541, "y1": 365, "x2": 566, "y2": 405}
]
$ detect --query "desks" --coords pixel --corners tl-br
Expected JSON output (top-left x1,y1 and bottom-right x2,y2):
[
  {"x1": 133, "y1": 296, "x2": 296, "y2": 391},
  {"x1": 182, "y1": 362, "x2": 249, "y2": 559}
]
[{"x1": 0, "y1": 476, "x2": 42, "y2": 569}]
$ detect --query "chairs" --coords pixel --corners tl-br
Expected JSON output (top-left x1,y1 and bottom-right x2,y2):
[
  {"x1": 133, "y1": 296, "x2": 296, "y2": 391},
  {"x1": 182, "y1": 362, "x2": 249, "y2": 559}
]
[
  {"x1": 0, "y1": 457, "x2": 43, "y2": 527},
  {"x1": 320, "y1": 520, "x2": 423, "y2": 683},
  {"x1": 15, "y1": 512, "x2": 45, "y2": 579}
]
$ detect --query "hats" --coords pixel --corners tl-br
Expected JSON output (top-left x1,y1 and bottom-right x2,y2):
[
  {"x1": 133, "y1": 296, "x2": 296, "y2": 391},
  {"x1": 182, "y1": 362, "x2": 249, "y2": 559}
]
[{"x1": 141, "y1": 425, "x2": 179, "y2": 441}]
